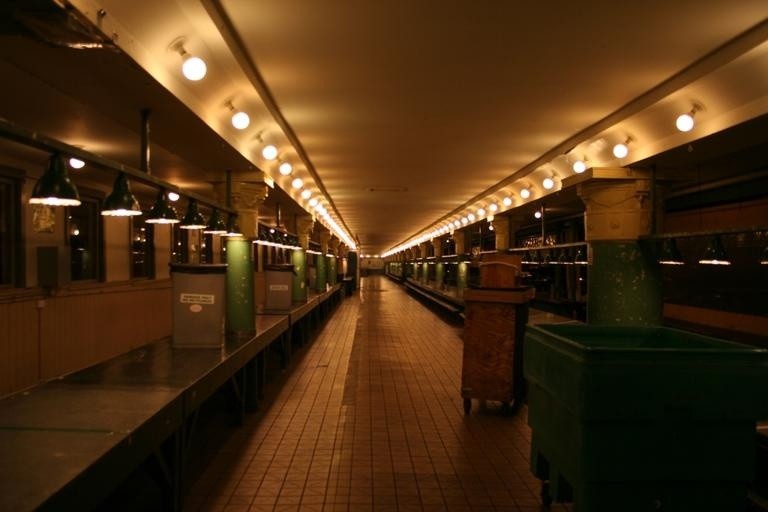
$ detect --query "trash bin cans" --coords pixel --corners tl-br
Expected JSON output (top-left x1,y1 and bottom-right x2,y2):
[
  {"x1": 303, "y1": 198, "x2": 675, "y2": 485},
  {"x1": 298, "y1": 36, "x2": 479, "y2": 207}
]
[
  {"x1": 169, "y1": 262, "x2": 229, "y2": 349},
  {"x1": 308, "y1": 264, "x2": 317, "y2": 288},
  {"x1": 520, "y1": 323, "x2": 766, "y2": 511},
  {"x1": 264, "y1": 264, "x2": 298, "y2": 314},
  {"x1": 461, "y1": 287, "x2": 536, "y2": 416}
]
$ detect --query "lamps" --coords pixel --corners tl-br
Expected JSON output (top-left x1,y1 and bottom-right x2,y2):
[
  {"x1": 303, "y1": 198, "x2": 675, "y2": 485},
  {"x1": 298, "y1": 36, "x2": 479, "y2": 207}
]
[
  {"x1": 28, "y1": 149, "x2": 84, "y2": 206},
  {"x1": 167, "y1": 36, "x2": 207, "y2": 82},
  {"x1": 223, "y1": 91, "x2": 251, "y2": 130},
  {"x1": 487, "y1": 99, "x2": 704, "y2": 214},
  {"x1": 145, "y1": 187, "x2": 181, "y2": 224},
  {"x1": 100, "y1": 169, "x2": 143, "y2": 217},
  {"x1": 179, "y1": 199, "x2": 242, "y2": 237}
]
